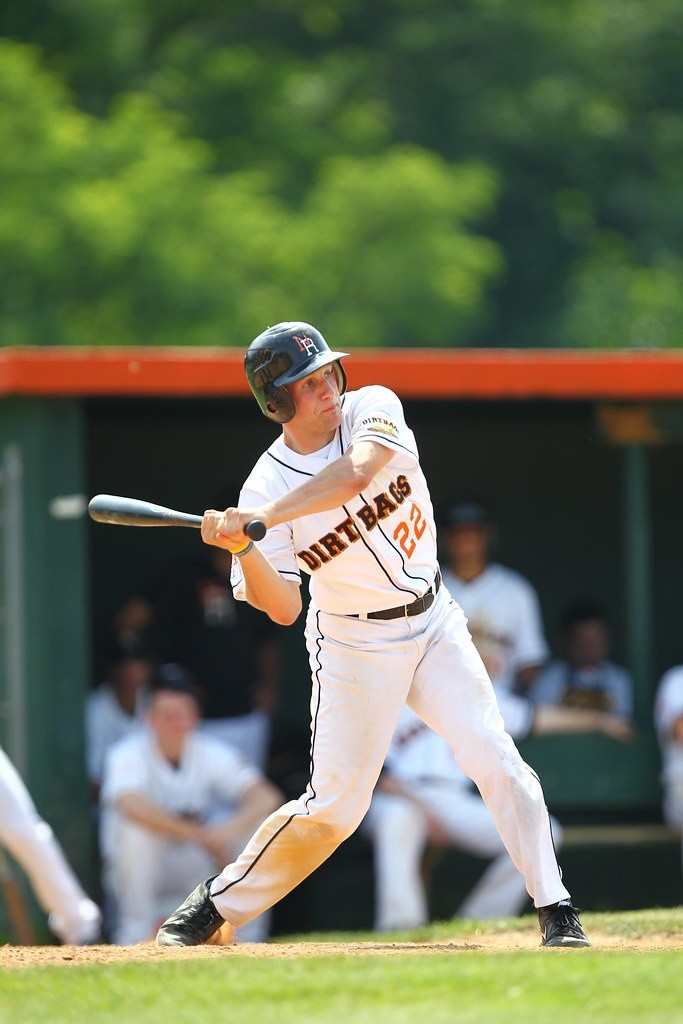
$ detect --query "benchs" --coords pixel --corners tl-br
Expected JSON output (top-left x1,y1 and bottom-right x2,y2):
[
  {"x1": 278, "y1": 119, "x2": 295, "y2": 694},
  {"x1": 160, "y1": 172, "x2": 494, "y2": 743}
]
[{"x1": 514, "y1": 717, "x2": 683, "y2": 848}]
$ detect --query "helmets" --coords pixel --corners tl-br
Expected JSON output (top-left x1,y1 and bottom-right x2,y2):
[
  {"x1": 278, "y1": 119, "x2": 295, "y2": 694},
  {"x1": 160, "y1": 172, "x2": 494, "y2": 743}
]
[{"x1": 243, "y1": 321, "x2": 351, "y2": 423}]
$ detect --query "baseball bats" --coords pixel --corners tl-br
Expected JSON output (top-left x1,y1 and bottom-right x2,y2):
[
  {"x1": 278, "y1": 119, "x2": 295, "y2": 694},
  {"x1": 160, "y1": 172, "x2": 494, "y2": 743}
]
[{"x1": 89, "y1": 494, "x2": 267, "y2": 542}]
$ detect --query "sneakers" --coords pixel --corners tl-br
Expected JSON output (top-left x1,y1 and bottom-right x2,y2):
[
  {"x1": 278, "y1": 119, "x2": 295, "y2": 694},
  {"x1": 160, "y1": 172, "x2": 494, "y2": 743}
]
[
  {"x1": 154, "y1": 873, "x2": 226, "y2": 948},
  {"x1": 538, "y1": 898, "x2": 592, "y2": 947}
]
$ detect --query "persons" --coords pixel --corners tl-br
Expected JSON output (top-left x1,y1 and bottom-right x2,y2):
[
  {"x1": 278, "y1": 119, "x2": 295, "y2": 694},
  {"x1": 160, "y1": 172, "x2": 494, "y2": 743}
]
[
  {"x1": 0, "y1": 746, "x2": 102, "y2": 947},
  {"x1": 86, "y1": 497, "x2": 683, "y2": 947},
  {"x1": 157, "y1": 321, "x2": 593, "y2": 947}
]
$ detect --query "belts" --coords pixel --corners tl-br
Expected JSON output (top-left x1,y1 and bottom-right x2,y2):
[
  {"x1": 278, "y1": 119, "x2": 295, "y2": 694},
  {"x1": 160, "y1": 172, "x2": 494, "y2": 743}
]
[{"x1": 344, "y1": 570, "x2": 441, "y2": 620}]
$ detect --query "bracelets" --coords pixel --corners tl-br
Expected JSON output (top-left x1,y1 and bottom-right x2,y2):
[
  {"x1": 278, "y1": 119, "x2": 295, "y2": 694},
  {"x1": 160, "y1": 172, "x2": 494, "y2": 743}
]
[{"x1": 235, "y1": 540, "x2": 253, "y2": 557}]
